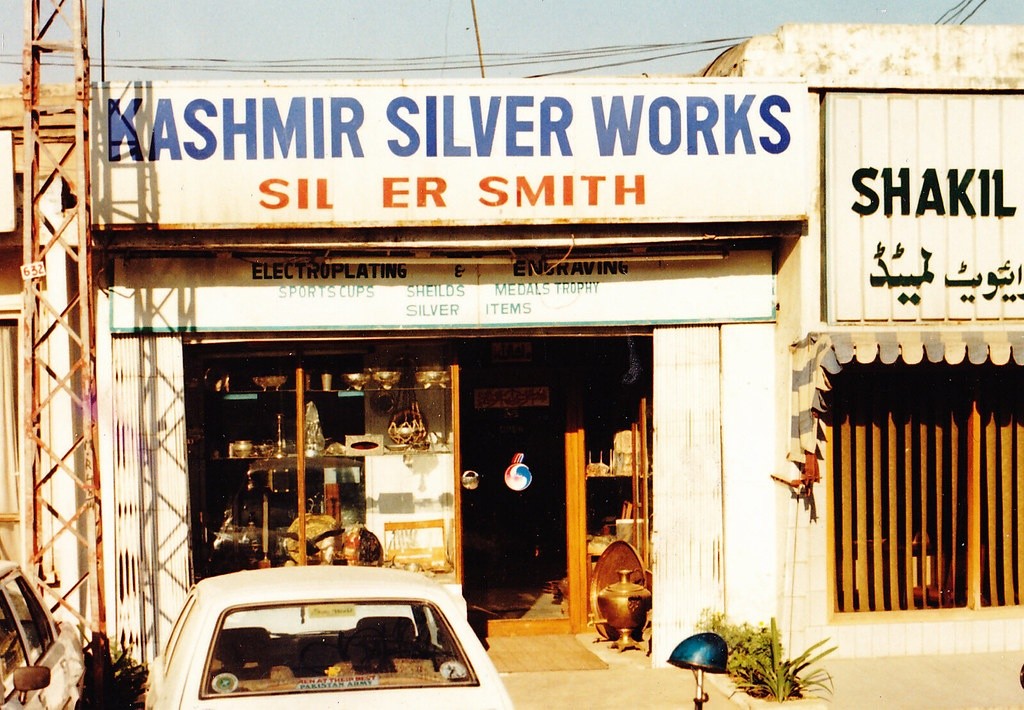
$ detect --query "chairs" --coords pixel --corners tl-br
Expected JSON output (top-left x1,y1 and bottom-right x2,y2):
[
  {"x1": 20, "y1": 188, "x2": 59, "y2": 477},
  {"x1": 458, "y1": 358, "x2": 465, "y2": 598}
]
[
  {"x1": 349, "y1": 616, "x2": 417, "y2": 671},
  {"x1": 207, "y1": 627, "x2": 273, "y2": 682}
]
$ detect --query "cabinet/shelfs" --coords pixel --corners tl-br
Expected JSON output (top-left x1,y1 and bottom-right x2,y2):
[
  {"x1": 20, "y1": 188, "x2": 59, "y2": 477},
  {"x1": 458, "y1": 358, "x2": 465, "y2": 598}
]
[{"x1": 191, "y1": 352, "x2": 463, "y2": 584}]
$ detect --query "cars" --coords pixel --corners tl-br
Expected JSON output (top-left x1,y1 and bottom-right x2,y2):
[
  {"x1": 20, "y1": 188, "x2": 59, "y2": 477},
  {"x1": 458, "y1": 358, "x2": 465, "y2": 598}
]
[
  {"x1": 147, "y1": 565, "x2": 516, "y2": 710},
  {"x1": 1, "y1": 560, "x2": 91, "y2": 710}
]
